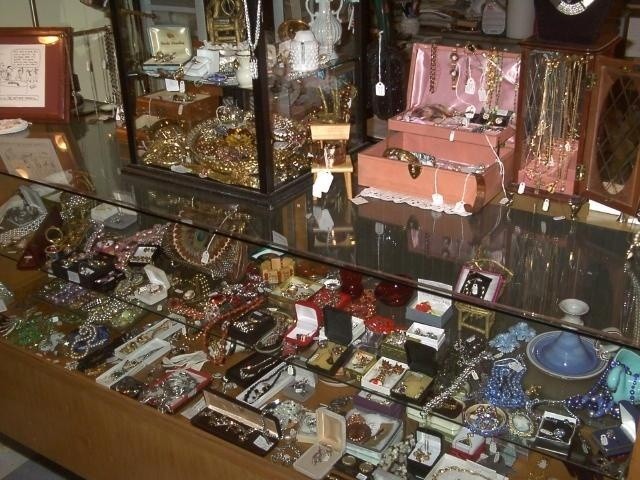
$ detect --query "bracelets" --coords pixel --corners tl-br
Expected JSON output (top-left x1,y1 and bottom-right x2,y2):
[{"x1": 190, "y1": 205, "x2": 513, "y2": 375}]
[
  {"x1": 408, "y1": 150, "x2": 437, "y2": 167},
  {"x1": 379, "y1": 146, "x2": 419, "y2": 167}
]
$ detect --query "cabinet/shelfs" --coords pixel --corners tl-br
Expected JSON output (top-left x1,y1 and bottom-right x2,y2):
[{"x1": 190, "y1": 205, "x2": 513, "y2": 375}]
[{"x1": 104, "y1": 1, "x2": 366, "y2": 210}]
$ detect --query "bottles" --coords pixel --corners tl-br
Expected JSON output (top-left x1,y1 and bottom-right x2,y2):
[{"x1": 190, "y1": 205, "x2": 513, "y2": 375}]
[{"x1": 235, "y1": 50, "x2": 253, "y2": 89}]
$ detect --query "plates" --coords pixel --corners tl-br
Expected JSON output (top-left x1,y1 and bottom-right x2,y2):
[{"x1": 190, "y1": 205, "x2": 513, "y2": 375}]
[{"x1": 525, "y1": 331, "x2": 610, "y2": 381}]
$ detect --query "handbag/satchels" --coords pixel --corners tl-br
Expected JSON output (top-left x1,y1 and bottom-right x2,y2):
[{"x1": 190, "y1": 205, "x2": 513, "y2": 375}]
[{"x1": 153, "y1": 204, "x2": 248, "y2": 285}]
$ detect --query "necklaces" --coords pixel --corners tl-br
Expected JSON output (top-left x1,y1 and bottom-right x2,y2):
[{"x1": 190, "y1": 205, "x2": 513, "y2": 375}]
[
  {"x1": 60, "y1": 25, "x2": 125, "y2": 129},
  {"x1": 242, "y1": 0, "x2": 263, "y2": 80},
  {"x1": 133, "y1": 79, "x2": 364, "y2": 189},
  {"x1": 2, "y1": 167, "x2": 639, "y2": 479},
  {"x1": 427, "y1": 38, "x2": 584, "y2": 202}
]
